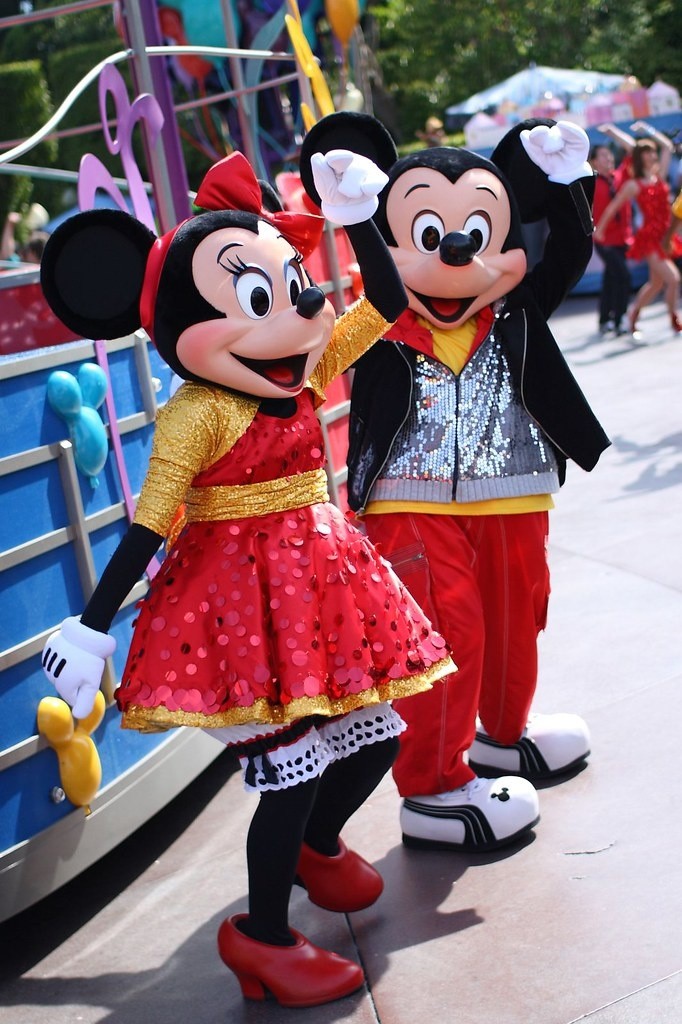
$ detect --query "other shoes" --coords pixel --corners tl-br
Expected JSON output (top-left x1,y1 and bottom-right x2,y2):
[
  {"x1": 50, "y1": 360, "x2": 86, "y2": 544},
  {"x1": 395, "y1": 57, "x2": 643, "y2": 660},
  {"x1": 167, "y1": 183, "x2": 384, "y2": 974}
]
[
  {"x1": 615, "y1": 328, "x2": 628, "y2": 336},
  {"x1": 627, "y1": 312, "x2": 638, "y2": 333},
  {"x1": 671, "y1": 314, "x2": 682, "y2": 332},
  {"x1": 600, "y1": 325, "x2": 613, "y2": 333}
]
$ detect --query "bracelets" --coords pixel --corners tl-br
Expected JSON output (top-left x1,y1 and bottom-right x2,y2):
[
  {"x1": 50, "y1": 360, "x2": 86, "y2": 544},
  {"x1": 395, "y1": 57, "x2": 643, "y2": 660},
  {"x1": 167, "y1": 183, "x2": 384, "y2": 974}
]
[{"x1": 643, "y1": 125, "x2": 657, "y2": 137}]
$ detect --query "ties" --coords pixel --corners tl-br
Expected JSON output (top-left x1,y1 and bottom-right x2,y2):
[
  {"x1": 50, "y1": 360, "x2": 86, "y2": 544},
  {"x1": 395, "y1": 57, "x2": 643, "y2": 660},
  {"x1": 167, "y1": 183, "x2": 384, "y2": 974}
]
[{"x1": 598, "y1": 172, "x2": 621, "y2": 224}]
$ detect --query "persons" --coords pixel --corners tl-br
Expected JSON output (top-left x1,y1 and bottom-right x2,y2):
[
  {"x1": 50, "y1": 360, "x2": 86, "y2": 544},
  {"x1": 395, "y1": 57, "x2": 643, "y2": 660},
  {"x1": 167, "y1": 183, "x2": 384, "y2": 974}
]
[
  {"x1": 300, "y1": 111, "x2": 614, "y2": 853},
  {"x1": 589, "y1": 120, "x2": 682, "y2": 345},
  {"x1": 412, "y1": 113, "x2": 448, "y2": 150},
  {"x1": 41, "y1": 150, "x2": 459, "y2": 1008},
  {"x1": 0, "y1": 211, "x2": 50, "y2": 272}
]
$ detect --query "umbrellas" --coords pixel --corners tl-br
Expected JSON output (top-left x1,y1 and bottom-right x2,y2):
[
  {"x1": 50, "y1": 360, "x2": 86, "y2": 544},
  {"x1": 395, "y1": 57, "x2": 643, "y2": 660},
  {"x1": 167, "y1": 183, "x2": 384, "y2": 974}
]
[{"x1": 445, "y1": 62, "x2": 626, "y2": 114}]
